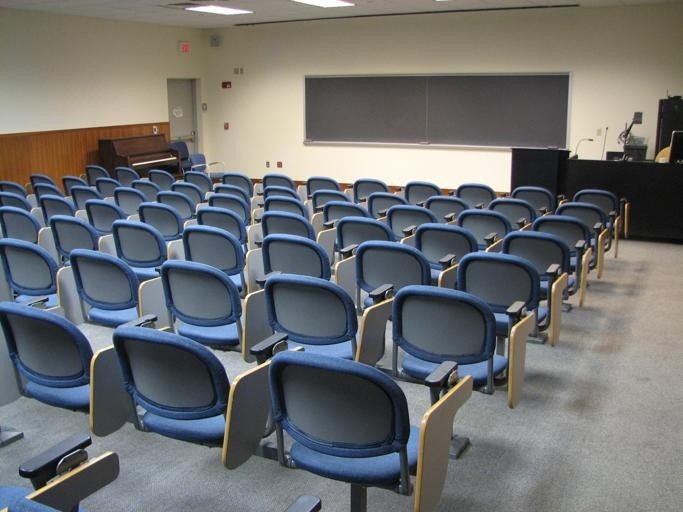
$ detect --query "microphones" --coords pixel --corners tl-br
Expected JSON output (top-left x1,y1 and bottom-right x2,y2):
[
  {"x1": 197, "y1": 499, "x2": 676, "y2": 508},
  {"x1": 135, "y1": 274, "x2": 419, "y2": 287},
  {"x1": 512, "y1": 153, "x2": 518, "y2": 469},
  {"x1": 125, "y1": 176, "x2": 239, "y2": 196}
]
[
  {"x1": 570, "y1": 138, "x2": 593, "y2": 159},
  {"x1": 601, "y1": 127, "x2": 609, "y2": 160}
]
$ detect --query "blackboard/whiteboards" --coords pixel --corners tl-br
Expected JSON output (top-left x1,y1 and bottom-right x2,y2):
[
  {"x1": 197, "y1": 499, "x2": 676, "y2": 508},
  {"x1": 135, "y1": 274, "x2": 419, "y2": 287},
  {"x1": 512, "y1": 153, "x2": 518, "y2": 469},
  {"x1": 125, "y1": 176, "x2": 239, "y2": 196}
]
[{"x1": 304, "y1": 72, "x2": 572, "y2": 151}]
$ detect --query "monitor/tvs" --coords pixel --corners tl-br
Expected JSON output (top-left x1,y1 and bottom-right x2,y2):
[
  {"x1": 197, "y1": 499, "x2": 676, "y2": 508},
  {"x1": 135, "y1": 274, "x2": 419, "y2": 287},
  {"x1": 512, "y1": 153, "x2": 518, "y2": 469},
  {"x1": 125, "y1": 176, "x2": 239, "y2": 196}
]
[{"x1": 668, "y1": 130, "x2": 683, "y2": 163}]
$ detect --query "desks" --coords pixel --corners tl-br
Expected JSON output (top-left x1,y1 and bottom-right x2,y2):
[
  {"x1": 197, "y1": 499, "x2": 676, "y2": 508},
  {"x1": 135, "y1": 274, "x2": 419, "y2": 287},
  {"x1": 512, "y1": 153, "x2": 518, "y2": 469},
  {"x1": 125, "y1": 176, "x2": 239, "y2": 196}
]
[{"x1": 559, "y1": 158, "x2": 682, "y2": 245}]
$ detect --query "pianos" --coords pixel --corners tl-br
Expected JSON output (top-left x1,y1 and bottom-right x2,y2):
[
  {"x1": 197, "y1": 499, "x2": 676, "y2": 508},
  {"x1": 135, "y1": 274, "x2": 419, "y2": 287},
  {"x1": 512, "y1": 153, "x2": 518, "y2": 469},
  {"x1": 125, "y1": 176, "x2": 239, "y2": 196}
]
[{"x1": 99, "y1": 133, "x2": 182, "y2": 181}]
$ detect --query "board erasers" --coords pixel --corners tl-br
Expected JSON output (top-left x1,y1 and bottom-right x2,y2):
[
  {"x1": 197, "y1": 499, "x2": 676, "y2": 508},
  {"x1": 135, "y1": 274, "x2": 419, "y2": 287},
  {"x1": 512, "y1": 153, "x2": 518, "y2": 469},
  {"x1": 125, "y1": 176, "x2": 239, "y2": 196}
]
[
  {"x1": 548, "y1": 145, "x2": 558, "y2": 149},
  {"x1": 305, "y1": 139, "x2": 312, "y2": 142}
]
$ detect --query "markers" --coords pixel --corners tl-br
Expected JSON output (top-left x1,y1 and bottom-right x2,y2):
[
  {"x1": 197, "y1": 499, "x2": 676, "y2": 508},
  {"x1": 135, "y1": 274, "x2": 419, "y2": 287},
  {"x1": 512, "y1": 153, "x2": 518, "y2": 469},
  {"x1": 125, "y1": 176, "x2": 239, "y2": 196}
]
[{"x1": 420, "y1": 141, "x2": 430, "y2": 144}]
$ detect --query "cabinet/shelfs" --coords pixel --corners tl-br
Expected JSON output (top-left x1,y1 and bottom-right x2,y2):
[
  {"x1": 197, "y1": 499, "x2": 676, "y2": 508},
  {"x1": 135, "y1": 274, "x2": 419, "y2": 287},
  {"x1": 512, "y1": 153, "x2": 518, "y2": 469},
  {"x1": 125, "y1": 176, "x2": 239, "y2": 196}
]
[{"x1": 655, "y1": 96, "x2": 683, "y2": 158}]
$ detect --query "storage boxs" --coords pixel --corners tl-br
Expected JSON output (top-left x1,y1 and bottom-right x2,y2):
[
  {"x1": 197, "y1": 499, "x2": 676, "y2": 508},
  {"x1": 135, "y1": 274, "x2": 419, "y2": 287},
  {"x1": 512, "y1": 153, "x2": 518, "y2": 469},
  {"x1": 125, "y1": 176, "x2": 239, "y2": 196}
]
[{"x1": 623, "y1": 145, "x2": 648, "y2": 162}]
